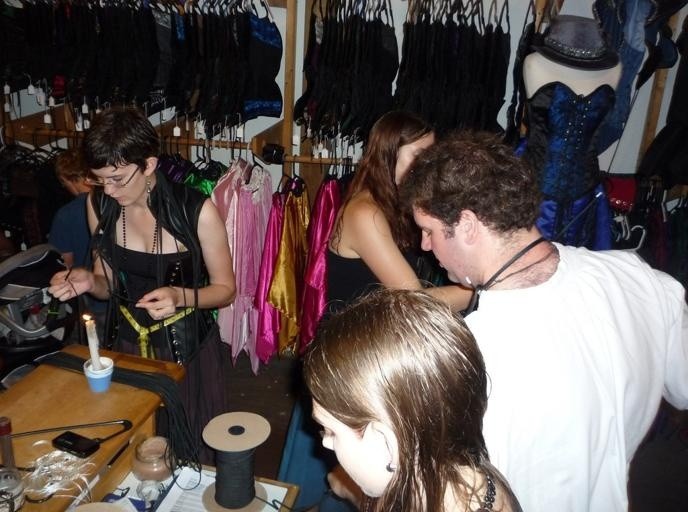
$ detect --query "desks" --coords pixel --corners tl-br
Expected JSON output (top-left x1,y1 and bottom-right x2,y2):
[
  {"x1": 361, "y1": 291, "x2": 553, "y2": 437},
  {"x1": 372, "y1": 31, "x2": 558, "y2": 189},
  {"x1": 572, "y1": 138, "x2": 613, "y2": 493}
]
[{"x1": 0, "y1": 340, "x2": 299, "y2": 512}]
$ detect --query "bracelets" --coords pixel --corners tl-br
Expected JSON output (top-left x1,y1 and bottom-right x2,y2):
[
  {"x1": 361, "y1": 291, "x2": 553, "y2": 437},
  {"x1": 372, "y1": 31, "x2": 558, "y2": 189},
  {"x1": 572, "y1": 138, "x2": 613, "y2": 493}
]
[
  {"x1": 193, "y1": 288, "x2": 202, "y2": 310},
  {"x1": 465, "y1": 287, "x2": 479, "y2": 312}
]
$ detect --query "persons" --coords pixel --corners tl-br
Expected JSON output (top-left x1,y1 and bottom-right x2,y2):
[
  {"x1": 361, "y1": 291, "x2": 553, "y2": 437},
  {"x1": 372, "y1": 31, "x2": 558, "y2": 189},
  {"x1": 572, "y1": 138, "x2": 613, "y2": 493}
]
[
  {"x1": 300, "y1": 288, "x2": 527, "y2": 512},
  {"x1": 274, "y1": 108, "x2": 478, "y2": 512},
  {"x1": 46, "y1": 148, "x2": 109, "y2": 350},
  {"x1": 323, "y1": 126, "x2": 688, "y2": 512},
  {"x1": 506, "y1": 6, "x2": 628, "y2": 253},
  {"x1": 44, "y1": 103, "x2": 239, "y2": 466}
]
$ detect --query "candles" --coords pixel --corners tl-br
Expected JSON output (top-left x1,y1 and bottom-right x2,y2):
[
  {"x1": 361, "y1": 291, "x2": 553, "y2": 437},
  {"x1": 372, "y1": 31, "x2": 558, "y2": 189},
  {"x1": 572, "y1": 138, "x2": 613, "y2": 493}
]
[{"x1": 85, "y1": 320, "x2": 104, "y2": 371}]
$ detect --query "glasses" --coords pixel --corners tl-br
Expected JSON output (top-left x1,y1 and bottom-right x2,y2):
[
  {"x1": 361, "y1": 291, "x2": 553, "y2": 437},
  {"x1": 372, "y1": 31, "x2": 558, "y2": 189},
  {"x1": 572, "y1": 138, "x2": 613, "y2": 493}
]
[{"x1": 83, "y1": 165, "x2": 143, "y2": 189}]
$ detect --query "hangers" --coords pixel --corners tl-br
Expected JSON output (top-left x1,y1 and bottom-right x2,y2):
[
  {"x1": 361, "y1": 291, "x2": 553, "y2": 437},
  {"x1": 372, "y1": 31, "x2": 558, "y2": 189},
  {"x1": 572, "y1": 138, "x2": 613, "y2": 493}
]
[
  {"x1": 0, "y1": 123, "x2": 363, "y2": 197},
  {"x1": 99, "y1": 0, "x2": 504, "y2": 36}
]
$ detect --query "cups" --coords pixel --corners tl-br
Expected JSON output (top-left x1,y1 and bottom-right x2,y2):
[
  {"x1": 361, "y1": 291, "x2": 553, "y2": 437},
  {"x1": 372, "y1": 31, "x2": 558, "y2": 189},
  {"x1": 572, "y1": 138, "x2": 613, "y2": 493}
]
[{"x1": 82, "y1": 356, "x2": 115, "y2": 395}]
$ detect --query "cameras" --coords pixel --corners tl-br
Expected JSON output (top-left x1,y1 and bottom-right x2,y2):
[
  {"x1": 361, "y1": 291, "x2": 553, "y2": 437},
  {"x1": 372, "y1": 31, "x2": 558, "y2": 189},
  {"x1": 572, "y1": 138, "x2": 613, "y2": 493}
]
[{"x1": 52, "y1": 431, "x2": 100, "y2": 459}]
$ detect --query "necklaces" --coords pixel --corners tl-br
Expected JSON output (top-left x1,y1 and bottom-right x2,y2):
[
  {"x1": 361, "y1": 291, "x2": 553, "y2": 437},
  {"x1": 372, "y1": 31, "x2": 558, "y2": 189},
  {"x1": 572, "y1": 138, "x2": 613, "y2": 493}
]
[
  {"x1": 477, "y1": 466, "x2": 497, "y2": 512},
  {"x1": 119, "y1": 204, "x2": 158, "y2": 254},
  {"x1": 487, "y1": 250, "x2": 557, "y2": 290},
  {"x1": 483, "y1": 235, "x2": 547, "y2": 291}
]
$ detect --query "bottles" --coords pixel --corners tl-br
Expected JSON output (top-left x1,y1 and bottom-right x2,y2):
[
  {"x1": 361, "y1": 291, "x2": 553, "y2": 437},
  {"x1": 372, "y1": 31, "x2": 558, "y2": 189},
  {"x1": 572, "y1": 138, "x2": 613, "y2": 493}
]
[{"x1": 130, "y1": 435, "x2": 176, "y2": 484}]
